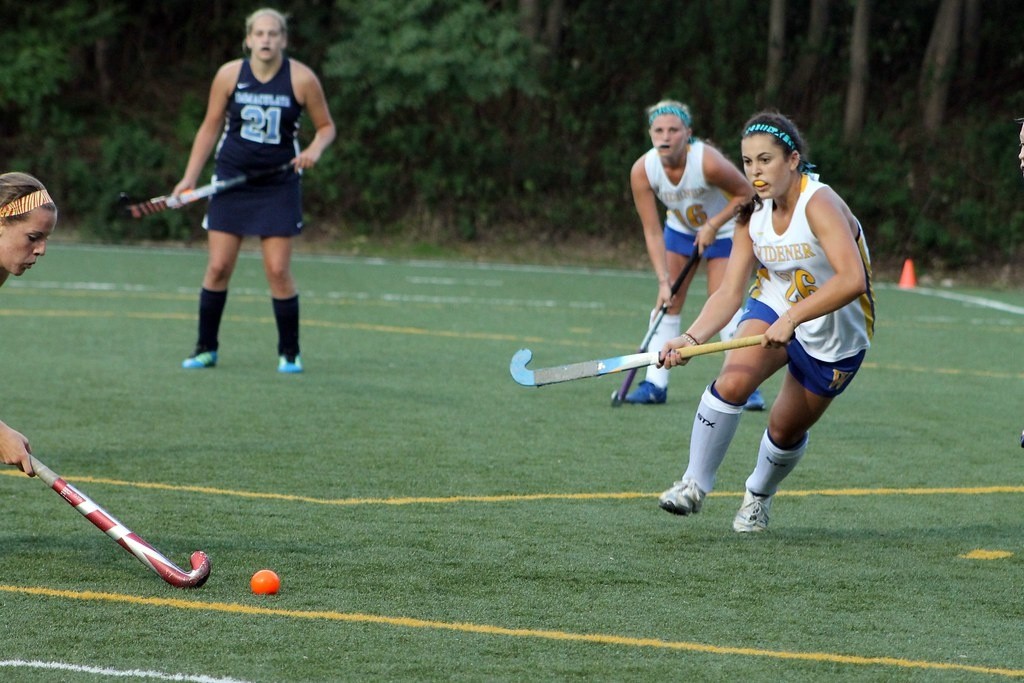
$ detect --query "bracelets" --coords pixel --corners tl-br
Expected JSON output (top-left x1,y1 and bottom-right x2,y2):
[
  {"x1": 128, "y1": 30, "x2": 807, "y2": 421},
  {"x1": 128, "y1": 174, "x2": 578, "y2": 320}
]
[{"x1": 681, "y1": 331, "x2": 700, "y2": 346}]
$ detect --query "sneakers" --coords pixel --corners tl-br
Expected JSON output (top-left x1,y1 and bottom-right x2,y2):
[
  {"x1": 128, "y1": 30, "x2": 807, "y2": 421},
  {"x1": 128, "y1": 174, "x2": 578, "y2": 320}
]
[
  {"x1": 182, "y1": 350, "x2": 217, "y2": 369},
  {"x1": 277, "y1": 351, "x2": 303, "y2": 373},
  {"x1": 657, "y1": 478, "x2": 706, "y2": 516},
  {"x1": 623, "y1": 380, "x2": 667, "y2": 404},
  {"x1": 733, "y1": 489, "x2": 772, "y2": 533},
  {"x1": 743, "y1": 389, "x2": 766, "y2": 411}
]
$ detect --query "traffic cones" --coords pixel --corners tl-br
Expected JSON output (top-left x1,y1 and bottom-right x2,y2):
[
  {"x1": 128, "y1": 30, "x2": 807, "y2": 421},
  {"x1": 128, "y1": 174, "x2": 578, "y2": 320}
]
[{"x1": 899, "y1": 259, "x2": 918, "y2": 289}]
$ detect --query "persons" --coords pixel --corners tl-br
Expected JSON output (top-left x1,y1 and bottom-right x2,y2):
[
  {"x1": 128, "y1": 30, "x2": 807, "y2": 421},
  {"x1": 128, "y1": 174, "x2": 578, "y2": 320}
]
[
  {"x1": 655, "y1": 116, "x2": 875, "y2": 534},
  {"x1": 172, "y1": 8, "x2": 336, "y2": 372},
  {"x1": 0, "y1": 172, "x2": 56, "y2": 476},
  {"x1": 625, "y1": 100, "x2": 765, "y2": 409}
]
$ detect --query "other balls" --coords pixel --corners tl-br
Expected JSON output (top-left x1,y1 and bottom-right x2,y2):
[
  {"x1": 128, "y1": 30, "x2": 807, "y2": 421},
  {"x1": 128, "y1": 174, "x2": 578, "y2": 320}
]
[{"x1": 251, "y1": 568, "x2": 282, "y2": 597}]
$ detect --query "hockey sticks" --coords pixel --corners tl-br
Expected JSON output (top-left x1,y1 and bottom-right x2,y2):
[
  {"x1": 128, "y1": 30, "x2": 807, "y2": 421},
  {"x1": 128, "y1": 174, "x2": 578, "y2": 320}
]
[
  {"x1": 610, "y1": 245, "x2": 698, "y2": 407},
  {"x1": 29, "y1": 455, "x2": 212, "y2": 589},
  {"x1": 118, "y1": 164, "x2": 293, "y2": 219},
  {"x1": 509, "y1": 330, "x2": 797, "y2": 388}
]
